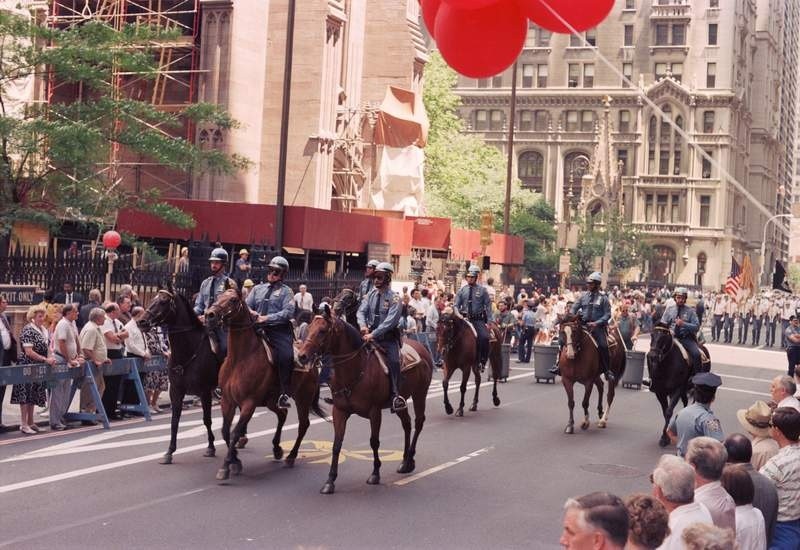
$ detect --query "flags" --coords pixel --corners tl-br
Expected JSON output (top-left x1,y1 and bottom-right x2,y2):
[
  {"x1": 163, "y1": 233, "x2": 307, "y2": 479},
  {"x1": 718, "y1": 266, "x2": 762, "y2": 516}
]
[
  {"x1": 739, "y1": 256, "x2": 754, "y2": 289},
  {"x1": 725, "y1": 257, "x2": 742, "y2": 296},
  {"x1": 772, "y1": 259, "x2": 793, "y2": 295}
]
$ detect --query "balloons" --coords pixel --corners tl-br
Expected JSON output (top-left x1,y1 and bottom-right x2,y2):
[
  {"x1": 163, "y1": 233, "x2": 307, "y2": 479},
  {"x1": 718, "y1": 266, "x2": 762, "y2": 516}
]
[{"x1": 418, "y1": 0, "x2": 615, "y2": 79}]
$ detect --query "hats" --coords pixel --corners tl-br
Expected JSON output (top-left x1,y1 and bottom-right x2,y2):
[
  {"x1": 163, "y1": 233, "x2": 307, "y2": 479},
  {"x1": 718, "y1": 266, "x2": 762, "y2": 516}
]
[
  {"x1": 692, "y1": 371, "x2": 723, "y2": 389},
  {"x1": 734, "y1": 399, "x2": 773, "y2": 438},
  {"x1": 789, "y1": 315, "x2": 799, "y2": 321},
  {"x1": 238, "y1": 248, "x2": 249, "y2": 256}
]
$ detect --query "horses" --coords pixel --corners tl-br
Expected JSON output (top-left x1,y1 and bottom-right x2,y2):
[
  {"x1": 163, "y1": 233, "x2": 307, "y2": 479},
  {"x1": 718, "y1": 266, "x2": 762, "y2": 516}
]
[
  {"x1": 646, "y1": 314, "x2": 711, "y2": 447},
  {"x1": 298, "y1": 303, "x2": 433, "y2": 495},
  {"x1": 556, "y1": 310, "x2": 627, "y2": 435},
  {"x1": 436, "y1": 305, "x2": 503, "y2": 417},
  {"x1": 333, "y1": 282, "x2": 364, "y2": 334},
  {"x1": 136, "y1": 276, "x2": 247, "y2": 465},
  {"x1": 205, "y1": 278, "x2": 331, "y2": 480}
]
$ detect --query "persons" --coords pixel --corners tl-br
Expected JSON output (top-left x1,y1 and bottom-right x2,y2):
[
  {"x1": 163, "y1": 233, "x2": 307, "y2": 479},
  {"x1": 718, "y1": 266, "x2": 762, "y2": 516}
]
[
  {"x1": 0, "y1": 296, "x2": 18, "y2": 431},
  {"x1": 10, "y1": 305, "x2": 56, "y2": 433},
  {"x1": 395, "y1": 263, "x2": 799, "y2": 366},
  {"x1": 143, "y1": 322, "x2": 171, "y2": 415},
  {"x1": 356, "y1": 259, "x2": 381, "y2": 301},
  {"x1": 119, "y1": 305, "x2": 152, "y2": 419},
  {"x1": 34, "y1": 238, "x2": 145, "y2": 340},
  {"x1": 559, "y1": 367, "x2": 800, "y2": 550},
  {"x1": 355, "y1": 261, "x2": 408, "y2": 414},
  {"x1": 191, "y1": 246, "x2": 239, "y2": 355},
  {"x1": 76, "y1": 309, "x2": 113, "y2": 427},
  {"x1": 96, "y1": 302, "x2": 130, "y2": 423},
  {"x1": 171, "y1": 248, "x2": 347, "y2": 341},
  {"x1": 245, "y1": 256, "x2": 297, "y2": 408},
  {"x1": 49, "y1": 306, "x2": 85, "y2": 431}
]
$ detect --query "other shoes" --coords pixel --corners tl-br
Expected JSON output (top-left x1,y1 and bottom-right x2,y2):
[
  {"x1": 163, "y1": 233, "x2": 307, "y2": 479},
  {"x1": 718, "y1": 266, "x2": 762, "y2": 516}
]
[
  {"x1": 20, "y1": 424, "x2": 37, "y2": 433},
  {"x1": 29, "y1": 423, "x2": 39, "y2": 430},
  {"x1": 51, "y1": 424, "x2": 66, "y2": 430}
]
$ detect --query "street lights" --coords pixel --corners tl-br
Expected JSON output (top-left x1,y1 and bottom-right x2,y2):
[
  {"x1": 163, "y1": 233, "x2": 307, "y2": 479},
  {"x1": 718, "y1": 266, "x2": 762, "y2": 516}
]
[{"x1": 757, "y1": 212, "x2": 795, "y2": 288}]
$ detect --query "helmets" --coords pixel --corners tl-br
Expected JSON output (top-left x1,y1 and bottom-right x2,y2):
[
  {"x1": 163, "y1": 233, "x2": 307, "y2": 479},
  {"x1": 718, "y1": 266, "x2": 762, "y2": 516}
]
[
  {"x1": 671, "y1": 286, "x2": 688, "y2": 299},
  {"x1": 374, "y1": 262, "x2": 395, "y2": 275},
  {"x1": 268, "y1": 256, "x2": 290, "y2": 274},
  {"x1": 465, "y1": 267, "x2": 480, "y2": 277},
  {"x1": 583, "y1": 271, "x2": 602, "y2": 285},
  {"x1": 367, "y1": 260, "x2": 380, "y2": 268},
  {"x1": 208, "y1": 249, "x2": 230, "y2": 264}
]
